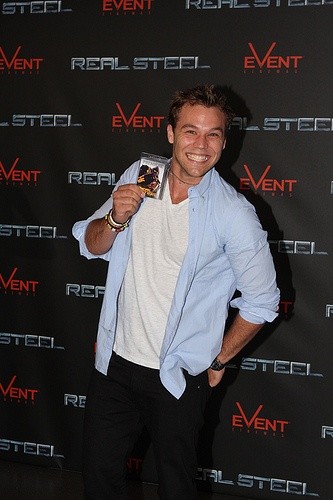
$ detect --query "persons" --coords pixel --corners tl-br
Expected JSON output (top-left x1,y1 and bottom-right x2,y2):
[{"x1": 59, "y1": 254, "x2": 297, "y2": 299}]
[{"x1": 71, "y1": 87, "x2": 281, "y2": 500}]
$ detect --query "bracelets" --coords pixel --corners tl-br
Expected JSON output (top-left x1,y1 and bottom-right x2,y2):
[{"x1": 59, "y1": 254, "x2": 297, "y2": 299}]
[{"x1": 104, "y1": 209, "x2": 131, "y2": 233}]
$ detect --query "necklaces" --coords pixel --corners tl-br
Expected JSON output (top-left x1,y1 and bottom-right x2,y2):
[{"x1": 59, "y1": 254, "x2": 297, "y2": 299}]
[{"x1": 169, "y1": 167, "x2": 200, "y2": 185}]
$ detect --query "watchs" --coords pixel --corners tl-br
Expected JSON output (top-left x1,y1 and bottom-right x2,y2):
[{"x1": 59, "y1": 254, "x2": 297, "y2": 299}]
[{"x1": 209, "y1": 357, "x2": 227, "y2": 371}]
